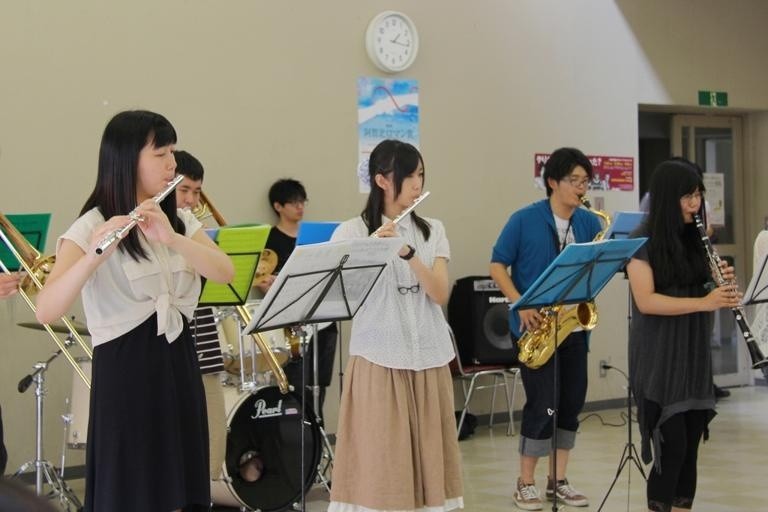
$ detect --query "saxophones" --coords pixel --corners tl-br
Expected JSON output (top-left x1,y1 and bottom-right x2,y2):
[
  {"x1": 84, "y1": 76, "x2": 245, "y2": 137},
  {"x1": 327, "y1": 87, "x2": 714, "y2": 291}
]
[{"x1": 518, "y1": 193, "x2": 610, "y2": 369}]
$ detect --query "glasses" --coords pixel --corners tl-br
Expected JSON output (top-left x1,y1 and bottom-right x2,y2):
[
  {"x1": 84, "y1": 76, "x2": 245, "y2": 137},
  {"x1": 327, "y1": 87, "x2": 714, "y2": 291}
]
[{"x1": 551, "y1": 173, "x2": 593, "y2": 190}]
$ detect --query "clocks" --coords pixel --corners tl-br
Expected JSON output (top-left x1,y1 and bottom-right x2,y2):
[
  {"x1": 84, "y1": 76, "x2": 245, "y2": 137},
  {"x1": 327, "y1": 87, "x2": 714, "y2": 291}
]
[{"x1": 366, "y1": 11, "x2": 420, "y2": 74}]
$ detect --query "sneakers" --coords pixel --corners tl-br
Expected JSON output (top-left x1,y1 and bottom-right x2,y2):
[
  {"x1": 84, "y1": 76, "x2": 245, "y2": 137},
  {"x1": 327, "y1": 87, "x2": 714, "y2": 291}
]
[
  {"x1": 512, "y1": 475, "x2": 545, "y2": 510},
  {"x1": 544, "y1": 473, "x2": 590, "y2": 507}
]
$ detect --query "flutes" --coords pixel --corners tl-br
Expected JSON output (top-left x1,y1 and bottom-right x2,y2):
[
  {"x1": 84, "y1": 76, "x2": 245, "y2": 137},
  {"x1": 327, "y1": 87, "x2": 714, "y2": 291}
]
[
  {"x1": 368, "y1": 190, "x2": 431, "y2": 240},
  {"x1": 96, "y1": 173, "x2": 184, "y2": 256}
]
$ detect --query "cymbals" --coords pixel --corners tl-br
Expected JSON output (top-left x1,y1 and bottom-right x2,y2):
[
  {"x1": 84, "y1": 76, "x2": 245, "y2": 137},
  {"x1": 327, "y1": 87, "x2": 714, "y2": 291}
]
[{"x1": 18, "y1": 319, "x2": 90, "y2": 335}]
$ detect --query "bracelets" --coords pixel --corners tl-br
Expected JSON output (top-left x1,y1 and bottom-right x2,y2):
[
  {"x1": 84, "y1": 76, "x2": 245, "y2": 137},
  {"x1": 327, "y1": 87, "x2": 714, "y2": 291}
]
[{"x1": 400, "y1": 245, "x2": 416, "y2": 260}]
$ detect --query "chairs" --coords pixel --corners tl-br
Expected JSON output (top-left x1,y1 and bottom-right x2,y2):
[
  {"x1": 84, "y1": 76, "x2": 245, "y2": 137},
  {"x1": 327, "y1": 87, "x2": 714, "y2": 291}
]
[
  {"x1": 489, "y1": 362, "x2": 528, "y2": 437},
  {"x1": 440, "y1": 321, "x2": 517, "y2": 437}
]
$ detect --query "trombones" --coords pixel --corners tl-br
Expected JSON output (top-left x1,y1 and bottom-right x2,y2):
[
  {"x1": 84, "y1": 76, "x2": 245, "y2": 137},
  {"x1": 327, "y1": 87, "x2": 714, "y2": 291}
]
[{"x1": 0, "y1": 210, "x2": 93, "y2": 388}]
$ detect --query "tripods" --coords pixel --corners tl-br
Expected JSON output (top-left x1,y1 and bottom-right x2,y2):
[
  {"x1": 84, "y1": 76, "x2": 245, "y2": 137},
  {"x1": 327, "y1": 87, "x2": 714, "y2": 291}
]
[
  {"x1": 1, "y1": 392, "x2": 80, "y2": 511},
  {"x1": 598, "y1": 296, "x2": 651, "y2": 512}
]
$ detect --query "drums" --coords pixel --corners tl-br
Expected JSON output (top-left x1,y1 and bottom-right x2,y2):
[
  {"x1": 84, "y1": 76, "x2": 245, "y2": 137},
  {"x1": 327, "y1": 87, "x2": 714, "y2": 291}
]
[
  {"x1": 218, "y1": 299, "x2": 292, "y2": 378},
  {"x1": 69, "y1": 357, "x2": 91, "y2": 450},
  {"x1": 210, "y1": 383, "x2": 323, "y2": 511}
]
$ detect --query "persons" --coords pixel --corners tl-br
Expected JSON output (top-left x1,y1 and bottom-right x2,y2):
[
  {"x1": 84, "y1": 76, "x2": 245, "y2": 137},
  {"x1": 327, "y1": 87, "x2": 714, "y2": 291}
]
[
  {"x1": 257, "y1": 179, "x2": 338, "y2": 427},
  {"x1": 0, "y1": 271, "x2": 22, "y2": 479},
  {"x1": 170, "y1": 149, "x2": 230, "y2": 481},
  {"x1": 490, "y1": 147, "x2": 601, "y2": 510},
  {"x1": 34, "y1": 110, "x2": 235, "y2": 512},
  {"x1": 329, "y1": 140, "x2": 465, "y2": 511},
  {"x1": 625, "y1": 160, "x2": 745, "y2": 512},
  {"x1": 640, "y1": 156, "x2": 733, "y2": 399}
]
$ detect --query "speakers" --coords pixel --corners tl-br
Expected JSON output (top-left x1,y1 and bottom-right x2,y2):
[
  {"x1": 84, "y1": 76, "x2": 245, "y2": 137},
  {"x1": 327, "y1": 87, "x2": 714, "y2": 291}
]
[{"x1": 447, "y1": 274, "x2": 518, "y2": 367}]
